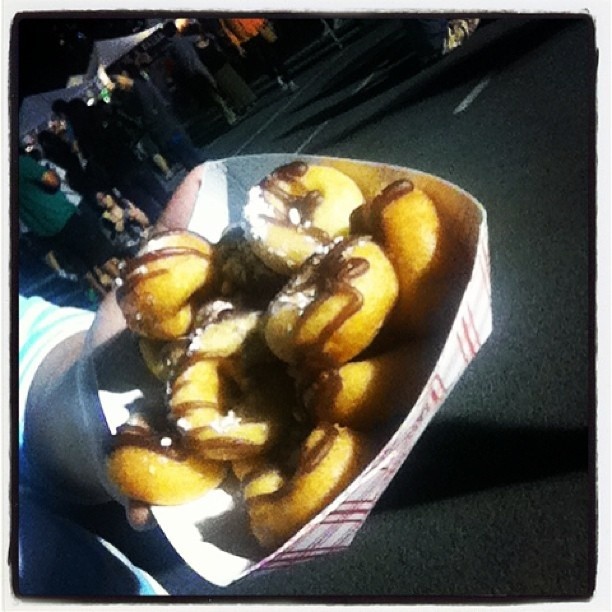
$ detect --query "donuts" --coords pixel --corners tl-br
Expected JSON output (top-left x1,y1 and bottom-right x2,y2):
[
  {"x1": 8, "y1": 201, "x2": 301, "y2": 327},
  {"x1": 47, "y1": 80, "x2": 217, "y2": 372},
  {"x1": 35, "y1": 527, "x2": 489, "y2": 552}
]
[{"x1": 104, "y1": 162, "x2": 449, "y2": 559}]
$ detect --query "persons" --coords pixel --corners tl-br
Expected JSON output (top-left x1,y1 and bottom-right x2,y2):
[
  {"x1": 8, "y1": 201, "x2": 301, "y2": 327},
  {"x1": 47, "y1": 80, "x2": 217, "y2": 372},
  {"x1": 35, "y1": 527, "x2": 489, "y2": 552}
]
[
  {"x1": 19, "y1": 163, "x2": 150, "y2": 301},
  {"x1": 20, "y1": 18, "x2": 296, "y2": 163},
  {"x1": 16, "y1": 161, "x2": 208, "y2": 596}
]
